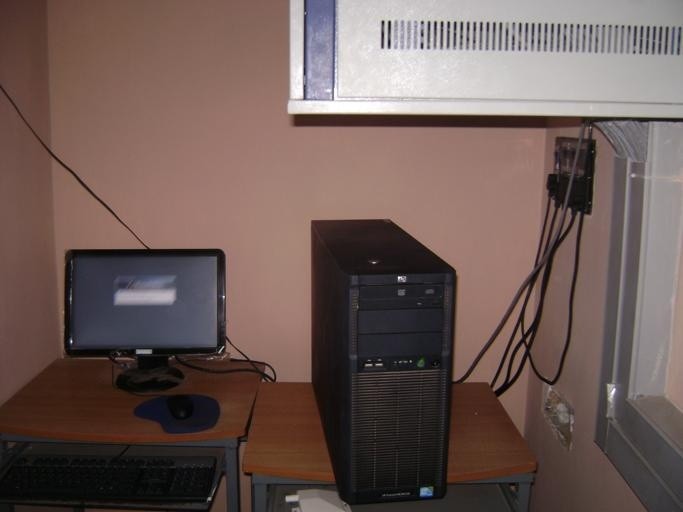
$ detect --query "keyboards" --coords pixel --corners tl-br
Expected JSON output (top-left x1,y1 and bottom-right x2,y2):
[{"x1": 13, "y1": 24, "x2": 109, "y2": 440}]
[{"x1": 0, "y1": 454, "x2": 217, "y2": 503}]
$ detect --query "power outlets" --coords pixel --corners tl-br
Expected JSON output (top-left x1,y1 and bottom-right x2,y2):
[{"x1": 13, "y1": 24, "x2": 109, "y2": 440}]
[{"x1": 549, "y1": 137, "x2": 596, "y2": 215}]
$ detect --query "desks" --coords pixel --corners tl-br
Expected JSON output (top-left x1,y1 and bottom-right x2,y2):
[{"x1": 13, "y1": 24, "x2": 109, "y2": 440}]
[
  {"x1": 244, "y1": 379, "x2": 537, "y2": 512},
  {"x1": 1, "y1": 359, "x2": 266, "y2": 512}
]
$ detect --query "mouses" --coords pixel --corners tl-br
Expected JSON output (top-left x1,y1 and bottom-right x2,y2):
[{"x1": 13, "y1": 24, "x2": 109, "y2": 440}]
[{"x1": 167, "y1": 395, "x2": 193, "y2": 418}]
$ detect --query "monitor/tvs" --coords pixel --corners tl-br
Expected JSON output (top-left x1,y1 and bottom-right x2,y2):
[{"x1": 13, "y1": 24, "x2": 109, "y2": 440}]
[{"x1": 63, "y1": 248, "x2": 227, "y2": 390}]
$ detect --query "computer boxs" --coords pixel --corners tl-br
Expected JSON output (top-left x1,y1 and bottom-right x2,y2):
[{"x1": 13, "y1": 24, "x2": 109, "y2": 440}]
[{"x1": 312, "y1": 217, "x2": 458, "y2": 505}]
